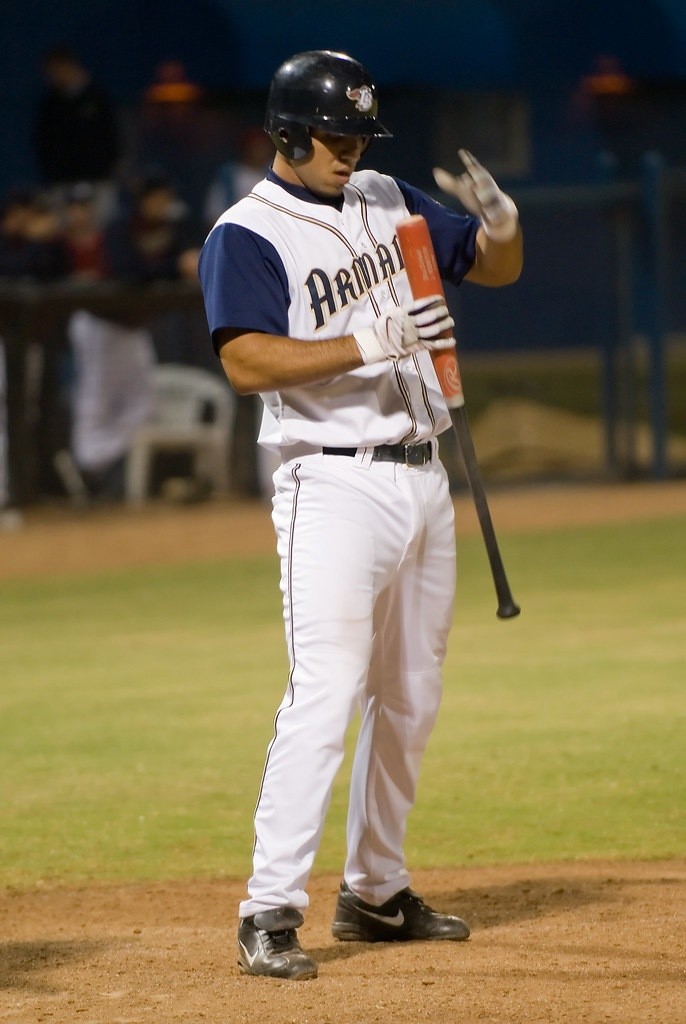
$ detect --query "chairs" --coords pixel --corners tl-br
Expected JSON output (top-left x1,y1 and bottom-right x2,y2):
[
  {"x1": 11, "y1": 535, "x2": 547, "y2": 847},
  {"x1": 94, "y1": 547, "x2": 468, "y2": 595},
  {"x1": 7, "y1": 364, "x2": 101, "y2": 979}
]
[{"x1": 68, "y1": 310, "x2": 233, "y2": 509}]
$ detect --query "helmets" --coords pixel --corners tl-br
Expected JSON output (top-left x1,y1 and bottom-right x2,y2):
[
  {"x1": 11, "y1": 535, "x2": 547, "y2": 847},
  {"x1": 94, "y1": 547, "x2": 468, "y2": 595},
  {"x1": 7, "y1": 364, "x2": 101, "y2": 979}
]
[{"x1": 262, "y1": 51, "x2": 394, "y2": 162}]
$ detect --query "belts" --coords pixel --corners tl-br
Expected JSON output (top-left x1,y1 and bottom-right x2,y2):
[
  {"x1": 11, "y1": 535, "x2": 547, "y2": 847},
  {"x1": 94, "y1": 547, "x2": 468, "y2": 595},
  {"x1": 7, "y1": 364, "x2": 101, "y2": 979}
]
[{"x1": 323, "y1": 442, "x2": 433, "y2": 467}]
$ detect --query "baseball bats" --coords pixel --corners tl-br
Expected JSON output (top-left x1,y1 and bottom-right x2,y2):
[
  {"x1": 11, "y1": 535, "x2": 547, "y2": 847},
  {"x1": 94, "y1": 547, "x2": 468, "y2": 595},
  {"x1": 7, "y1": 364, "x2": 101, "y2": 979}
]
[{"x1": 394, "y1": 213, "x2": 522, "y2": 620}]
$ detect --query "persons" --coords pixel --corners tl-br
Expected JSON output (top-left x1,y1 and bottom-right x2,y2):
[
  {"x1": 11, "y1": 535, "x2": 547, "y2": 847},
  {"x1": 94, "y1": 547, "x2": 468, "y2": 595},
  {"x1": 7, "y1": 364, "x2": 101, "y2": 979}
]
[
  {"x1": 198, "y1": 47, "x2": 525, "y2": 983},
  {"x1": 1, "y1": 51, "x2": 674, "y2": 287}
]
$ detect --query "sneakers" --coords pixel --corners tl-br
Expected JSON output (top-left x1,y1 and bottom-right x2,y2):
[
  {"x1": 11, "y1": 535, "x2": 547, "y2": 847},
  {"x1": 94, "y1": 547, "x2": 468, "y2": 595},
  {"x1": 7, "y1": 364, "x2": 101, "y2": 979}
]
[
  {"x1": 235, "y1": 907, "x2": 319, "y2": 980},
  {"x1": 331, "y1": 880, "x2": 471, "y2": 941}
]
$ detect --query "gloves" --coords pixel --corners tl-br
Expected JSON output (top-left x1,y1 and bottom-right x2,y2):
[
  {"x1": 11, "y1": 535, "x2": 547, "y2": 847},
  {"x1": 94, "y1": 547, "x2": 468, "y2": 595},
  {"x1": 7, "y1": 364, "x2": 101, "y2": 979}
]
[
  {"x1": 433, "y1": 150, "x2": 517, "y2": 242},
  {"x1": 351, "y1": 294, "x2": 457, "y2": 368}
]
